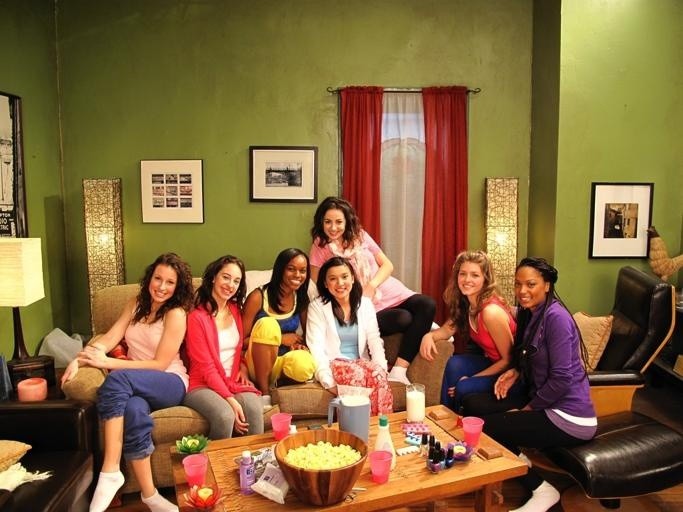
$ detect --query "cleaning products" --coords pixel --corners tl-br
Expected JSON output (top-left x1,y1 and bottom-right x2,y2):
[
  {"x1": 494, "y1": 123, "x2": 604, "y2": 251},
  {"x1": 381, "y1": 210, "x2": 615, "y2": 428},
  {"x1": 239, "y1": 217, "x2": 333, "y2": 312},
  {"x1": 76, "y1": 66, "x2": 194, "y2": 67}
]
[{"x1": 240, "y1": 451, "x2": 257, "y2": 496}]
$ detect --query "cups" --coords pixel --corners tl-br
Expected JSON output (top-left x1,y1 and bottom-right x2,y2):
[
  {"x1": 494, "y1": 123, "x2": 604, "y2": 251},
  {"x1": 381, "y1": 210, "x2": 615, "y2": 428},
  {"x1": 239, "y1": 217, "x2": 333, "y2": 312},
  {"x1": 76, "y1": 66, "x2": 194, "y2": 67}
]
[
  {"x1": 182, "y1": 454, "x2": 209, "y2": 487},
  {"x1": 405, "y1": 383, "x2": 424, "y2": 424},
  {"x1": 368, "y1": 450, "x2": 393, "y2": 485},
  {"x1": 16, "y1": 377, "x2": 47, "y2": 402},
  {"x1": 271, "y1": 413, "x2": 292, "y2": 440},
  {"x1": 460, "y1": 416, "x2": 485, "y2": 448},
  {"x1": 327, "y1": 394, "x2": 370, "y2": 446}
]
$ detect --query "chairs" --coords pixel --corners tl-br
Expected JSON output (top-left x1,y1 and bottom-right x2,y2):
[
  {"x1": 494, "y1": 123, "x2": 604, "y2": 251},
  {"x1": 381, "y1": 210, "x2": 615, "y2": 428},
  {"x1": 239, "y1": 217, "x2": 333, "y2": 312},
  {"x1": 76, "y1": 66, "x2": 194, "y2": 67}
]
[{"x1": 569, "y1": 264, "x2": 676, "y2": 417}]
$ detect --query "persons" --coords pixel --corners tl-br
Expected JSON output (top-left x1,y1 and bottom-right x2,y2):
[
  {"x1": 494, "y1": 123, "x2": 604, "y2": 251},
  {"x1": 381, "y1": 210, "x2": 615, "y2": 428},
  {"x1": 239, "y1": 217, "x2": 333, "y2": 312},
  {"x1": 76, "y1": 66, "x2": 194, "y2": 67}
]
[
  {"x1": 305, "y1": 257, "x2": 389, "y2": 397},
  {"x1": 60, "y1": 252, "x2": 190, "y2": 512},
  {"x1": 181, "y1": 255, "x2": 265, "y2": 441},
  {"x1": 461, "y1": 256, "x2": 599, "y2": 512},
  {"x1": 240, "y1": 247, "x2": 316, "y2": 409},
  {"x1": 309, "y1": 197, "x2": 436, "y2": 386},
  {"x1": 418, "y1": 251, "x2": 518, "y2": 411}
]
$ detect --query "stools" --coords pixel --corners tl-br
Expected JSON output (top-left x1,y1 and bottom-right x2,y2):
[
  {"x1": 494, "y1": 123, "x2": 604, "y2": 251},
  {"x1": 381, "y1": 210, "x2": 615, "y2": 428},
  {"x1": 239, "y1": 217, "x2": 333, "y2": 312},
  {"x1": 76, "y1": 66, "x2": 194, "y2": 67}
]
[{"x1": 514, "y1": 406, "x2": 682, "y2": 510}]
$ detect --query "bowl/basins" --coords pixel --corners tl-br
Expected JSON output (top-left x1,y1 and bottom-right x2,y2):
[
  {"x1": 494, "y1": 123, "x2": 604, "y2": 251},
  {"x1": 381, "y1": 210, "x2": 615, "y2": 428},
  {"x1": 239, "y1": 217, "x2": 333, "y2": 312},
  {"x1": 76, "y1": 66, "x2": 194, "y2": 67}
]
[{"x1": 273, "y1": 429, "x2": 369, "y2": 505}]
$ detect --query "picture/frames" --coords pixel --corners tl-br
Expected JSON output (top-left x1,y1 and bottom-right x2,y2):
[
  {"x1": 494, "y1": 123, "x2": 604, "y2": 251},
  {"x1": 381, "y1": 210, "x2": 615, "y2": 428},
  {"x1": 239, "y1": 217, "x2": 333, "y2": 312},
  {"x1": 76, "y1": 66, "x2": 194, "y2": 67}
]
[
  {"x1": 587, "y1": 180, "x2": 655, "y2": 261},
  {"x1": 247, "y1": 144, "x2": 318, "y2": 205},
  {"x1": 0, "y1": 90, "x2": 29, "y2": 239}
]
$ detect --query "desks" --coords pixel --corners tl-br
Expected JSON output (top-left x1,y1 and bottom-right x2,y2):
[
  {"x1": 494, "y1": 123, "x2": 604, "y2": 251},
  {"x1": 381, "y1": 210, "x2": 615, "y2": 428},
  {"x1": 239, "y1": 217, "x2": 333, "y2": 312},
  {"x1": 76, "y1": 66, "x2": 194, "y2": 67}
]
[
  {"x1": 3, "y1": 363, "x2": 68, "y2": 398},
  {"x1": 166, "y1": 402, "x2": 532, "y2": 512}
]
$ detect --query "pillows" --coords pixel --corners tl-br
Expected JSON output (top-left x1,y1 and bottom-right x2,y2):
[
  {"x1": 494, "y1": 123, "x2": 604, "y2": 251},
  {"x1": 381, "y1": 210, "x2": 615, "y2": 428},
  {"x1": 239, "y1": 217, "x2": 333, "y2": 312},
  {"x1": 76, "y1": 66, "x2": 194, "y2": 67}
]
[
  {"x1": 0, "y1": 438, "x2": 32, "y2": 474},
  {"x1": 572, "y1": 308, "x2": 614, "y2": 372}
]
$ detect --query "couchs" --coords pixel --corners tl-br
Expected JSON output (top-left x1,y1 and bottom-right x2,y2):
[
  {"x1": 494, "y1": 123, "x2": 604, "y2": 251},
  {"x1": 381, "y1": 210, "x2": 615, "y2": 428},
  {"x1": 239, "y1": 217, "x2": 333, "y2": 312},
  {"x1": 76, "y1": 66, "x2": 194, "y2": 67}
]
[
  {"x1": 0, "y1": 396, "x2": 99, "y2": 512},
  {"x1": 61, "y1": 269, "x2": 458, "y2": 508}
]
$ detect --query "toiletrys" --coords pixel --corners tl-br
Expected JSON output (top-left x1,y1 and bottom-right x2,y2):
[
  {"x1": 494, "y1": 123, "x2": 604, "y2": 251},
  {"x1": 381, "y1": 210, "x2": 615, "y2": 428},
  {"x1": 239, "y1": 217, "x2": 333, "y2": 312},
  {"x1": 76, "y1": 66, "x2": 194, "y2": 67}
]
[{"x1": 421, "y1": 433, "x2": 454, "y2": 473}]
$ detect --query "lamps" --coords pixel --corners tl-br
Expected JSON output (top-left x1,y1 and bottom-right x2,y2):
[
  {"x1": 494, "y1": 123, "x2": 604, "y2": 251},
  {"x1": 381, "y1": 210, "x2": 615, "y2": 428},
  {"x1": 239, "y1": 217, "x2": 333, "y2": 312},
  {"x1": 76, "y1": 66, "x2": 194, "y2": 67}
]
[
  {"x1": 481, "y1": 175, "x2": 520, "y2": 308},
  {"x1": 81, "y1": 176, "x2": 125, "y2": 336},
  {"x1": 0, "y1": 233, "x2": 55, "y2": 391}
]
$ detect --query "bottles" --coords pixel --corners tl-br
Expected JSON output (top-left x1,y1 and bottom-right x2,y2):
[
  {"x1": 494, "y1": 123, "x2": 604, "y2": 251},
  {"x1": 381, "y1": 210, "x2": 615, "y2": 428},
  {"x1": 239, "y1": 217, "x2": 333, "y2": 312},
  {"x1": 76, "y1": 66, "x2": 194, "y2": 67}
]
[
  {"x1": 419, "y1": 433, "x2": 454, "y2": 474},
  {"x1": 374, "y1": 415, "x2": 397, "y2": 471},
  {"x1": 238, "y1": 450, "x2": 259, "y2": 495}
]
